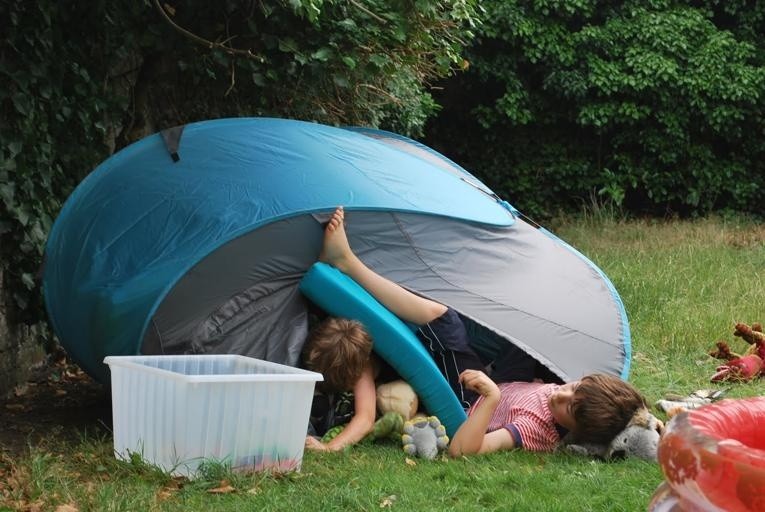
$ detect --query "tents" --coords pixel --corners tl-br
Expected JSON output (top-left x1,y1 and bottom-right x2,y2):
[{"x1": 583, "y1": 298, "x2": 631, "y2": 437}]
[{"x1": 41, "y1": 107, "x2": 627, "y2": 438}]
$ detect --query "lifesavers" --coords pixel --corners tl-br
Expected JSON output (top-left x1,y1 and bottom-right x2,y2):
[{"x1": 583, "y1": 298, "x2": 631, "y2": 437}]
[{"x1": 647, "y1": 395, "x2": 765, "y2": 512}]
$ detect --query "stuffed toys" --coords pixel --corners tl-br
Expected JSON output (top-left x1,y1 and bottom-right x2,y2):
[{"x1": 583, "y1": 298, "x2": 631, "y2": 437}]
[
  {"x1": 707, "y1": 321, "x2": 765, "y2": 382},
  {"x1": 566, "y1": 405, "x2": 667, "y2": 462},
  {"x1": 320, "y1": 378, "x2": 449, "y2": 459}
]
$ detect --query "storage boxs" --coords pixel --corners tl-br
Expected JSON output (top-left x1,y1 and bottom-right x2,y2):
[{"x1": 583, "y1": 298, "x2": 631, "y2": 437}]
[{"x1": 103, "y1": 354, "x2": 324, "y2": 483}]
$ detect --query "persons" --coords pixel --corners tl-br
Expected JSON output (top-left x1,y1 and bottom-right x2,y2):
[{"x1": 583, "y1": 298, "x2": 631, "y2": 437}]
[
  {"x1": 312, "y1": 202, "x2": 650, "y2": 457},
  {"x1": 299, "y1": 316, "x2": 378, "y2": 452}
]
